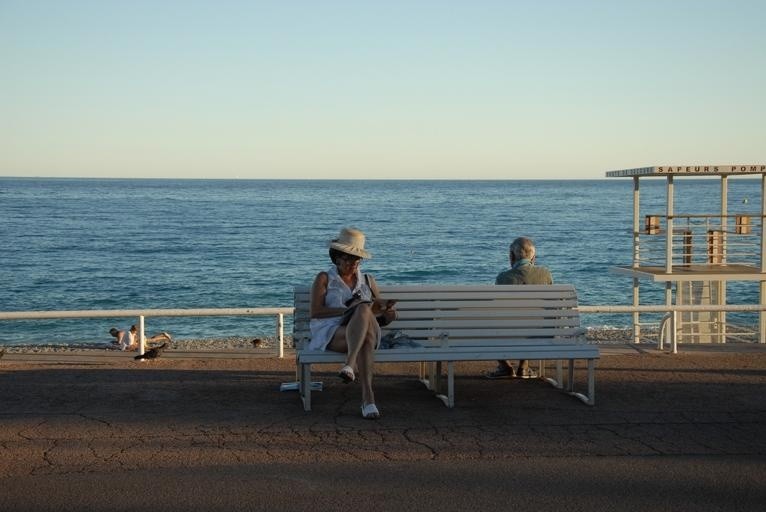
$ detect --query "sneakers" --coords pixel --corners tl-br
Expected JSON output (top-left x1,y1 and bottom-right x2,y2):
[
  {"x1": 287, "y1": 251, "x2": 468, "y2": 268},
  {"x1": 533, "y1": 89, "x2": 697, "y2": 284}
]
[
  {"x1": 484, "y1": 366, "x2": 516, "y2": 380},
  {"x1": 516, "y1": 368, "x2": 538, "y2": 378}
]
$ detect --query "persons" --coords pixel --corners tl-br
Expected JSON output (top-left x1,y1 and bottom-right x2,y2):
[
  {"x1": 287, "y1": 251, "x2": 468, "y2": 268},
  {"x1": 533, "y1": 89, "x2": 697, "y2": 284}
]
[
  {"x1": 117, "y1": 324, "x2": 138, "y2": 351},
  {"x1": 484, "y1": 237, "x2": 553, "y2": 379},
  {"x1": 308, "y1": 226, "x2": 400, "y2": 420},
  {"x1": 106, "y1": 327, "x2": 171, "y2": 350}
]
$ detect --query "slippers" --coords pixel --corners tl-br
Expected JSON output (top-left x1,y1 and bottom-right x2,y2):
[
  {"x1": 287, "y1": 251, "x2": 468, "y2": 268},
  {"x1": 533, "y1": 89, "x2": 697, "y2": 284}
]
[
  {"x1": 360, "y1": 403, "x2": 379, "y2": 418},
  {"x1": 338, "y1": 365, "x2": 355, "y2": 384}
]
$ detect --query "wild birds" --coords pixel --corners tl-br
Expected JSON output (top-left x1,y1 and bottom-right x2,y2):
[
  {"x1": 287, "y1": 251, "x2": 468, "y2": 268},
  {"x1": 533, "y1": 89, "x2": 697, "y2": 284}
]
[
  {"x1": 250, "y1": 338, "x2": 267, "y2": 347},
  {"x1": 135, "y1": 342, "x2": 169, "y2": 360}
]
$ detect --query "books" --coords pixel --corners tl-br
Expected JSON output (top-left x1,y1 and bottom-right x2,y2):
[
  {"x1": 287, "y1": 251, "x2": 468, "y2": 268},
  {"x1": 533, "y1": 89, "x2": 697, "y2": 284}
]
[
  {"x1": 279, "y1": 379, "x2": 324, "y2": 393},
  {"x1": 338, "y1": 282, "x2": 398, "y2": 328}
]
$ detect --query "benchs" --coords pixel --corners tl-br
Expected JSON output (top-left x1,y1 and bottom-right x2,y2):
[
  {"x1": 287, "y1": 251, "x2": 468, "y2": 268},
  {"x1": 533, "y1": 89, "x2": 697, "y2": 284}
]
[{"x1": 292, "y1": 283, "x2": 601, "y2": 411}]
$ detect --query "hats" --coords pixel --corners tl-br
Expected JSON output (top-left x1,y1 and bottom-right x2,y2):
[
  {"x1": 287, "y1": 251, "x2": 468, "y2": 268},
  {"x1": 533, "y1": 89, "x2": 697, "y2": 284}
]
[{"x1": 327, "y1": 228, "x2": 372, "y2": 259}]
